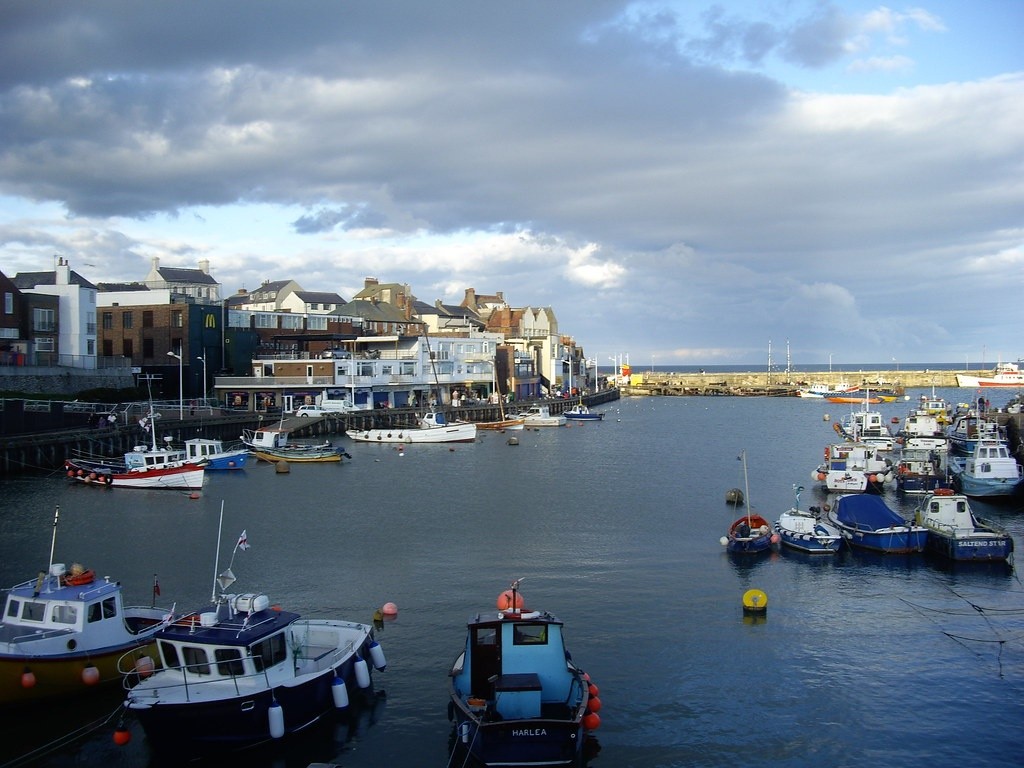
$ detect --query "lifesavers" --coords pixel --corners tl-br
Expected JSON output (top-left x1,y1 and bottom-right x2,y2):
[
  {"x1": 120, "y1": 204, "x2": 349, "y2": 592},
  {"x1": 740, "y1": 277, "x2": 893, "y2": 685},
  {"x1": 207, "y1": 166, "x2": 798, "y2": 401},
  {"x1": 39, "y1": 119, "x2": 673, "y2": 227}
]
[
  {"x1": 60, "y1": 569, "x2": 94, "y2": 586},
  {"x1": 378, "y1": 436, "x2": 381, "y2": 440},
  {"x1": 418, "y1": 421, "x2": 422, "y2": 426},
  {"x1": 388, "y1": 434, "x2": 391, "y2": 437},
  {"x1": 105, "y1": 475, "x2": 112, "y2": 485},
  {"x1": 399, "y1": 435, "x2": 402, "y2": 438},
  {"x1": 207, "y1": 460, "x2": 212, "y2": 466},
  {"x1": 365, "y1": 435, "x2": 368, "y2": 438},
  {"x1": 933, "y1": 489, "x2": 955, "y2": 496}
]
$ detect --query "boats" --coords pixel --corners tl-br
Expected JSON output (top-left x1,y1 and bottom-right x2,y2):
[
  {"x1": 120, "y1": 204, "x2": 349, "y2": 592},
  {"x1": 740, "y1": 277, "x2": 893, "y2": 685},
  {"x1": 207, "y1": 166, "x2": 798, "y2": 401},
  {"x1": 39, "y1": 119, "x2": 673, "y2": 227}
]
[
  {"x1": 913, "y1": 489, "x2": 1013, "y2": 561},
  {"x1": 346, "y1": 422, "x2": 476, "y2": 443},
  {"x1": 955, "y1": 363, "x2": 1024, "y2": 388},
  {"x1": 0, "y1": 504, "x2": 181, "y2": 713},
  {"x1": 773, "y1": 483, "x2": 842, "y2": 552},
  {"x1": 239, "y1": 418, "x2": 352, "y2": 461},
  {"x1": 116, "y1": 499, "x2": 386, "y2": 748},
  {"x1": 448, "y1": 576, "x2": 601, "y2": 768},
  {"x1": 796, "y1": 383, "x2": 1024, "y2": 496},
  {"x1": 504, "y1": 403, "x2": 606, "y2": 426},
  {"x1": 417, "y1": 413, "x2": 525, "y2": 431},
  {"x1": 719, "y1": 449, "x2": 774, "y2": 562},
  {"x1": 183, "y1": 438, "x2": 248, "y2": 469},
  {"x1": 827, "y1": 493, "x2": 930, "y2": 552},
  {"x1": 64, "y1": 436, "x2": 208, "y2": 490}
]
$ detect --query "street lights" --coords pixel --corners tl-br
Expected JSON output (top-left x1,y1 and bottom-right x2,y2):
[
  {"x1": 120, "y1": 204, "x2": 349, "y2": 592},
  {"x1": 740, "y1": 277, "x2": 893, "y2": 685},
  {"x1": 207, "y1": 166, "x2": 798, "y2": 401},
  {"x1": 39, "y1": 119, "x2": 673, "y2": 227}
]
[
  {"x1": 197, "y1": 357, "x2": 206, "y2": 406},
  {"x1": 168, "y1": 351, "x2": 182, "y2": 420},
  {"x1": 561, "y1": 358, "x2": 617, "y2": 397}
]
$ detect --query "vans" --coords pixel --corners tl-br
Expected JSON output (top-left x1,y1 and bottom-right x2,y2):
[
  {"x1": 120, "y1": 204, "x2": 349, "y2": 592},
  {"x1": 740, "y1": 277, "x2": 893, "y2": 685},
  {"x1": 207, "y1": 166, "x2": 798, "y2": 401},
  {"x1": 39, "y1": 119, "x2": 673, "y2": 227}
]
[
  {"x1": 321, "y1": 400, "x2": 361, "y2": 414},
  {"x1": 296, "y1": 405, "x2": 333, "y2": 418}
]
